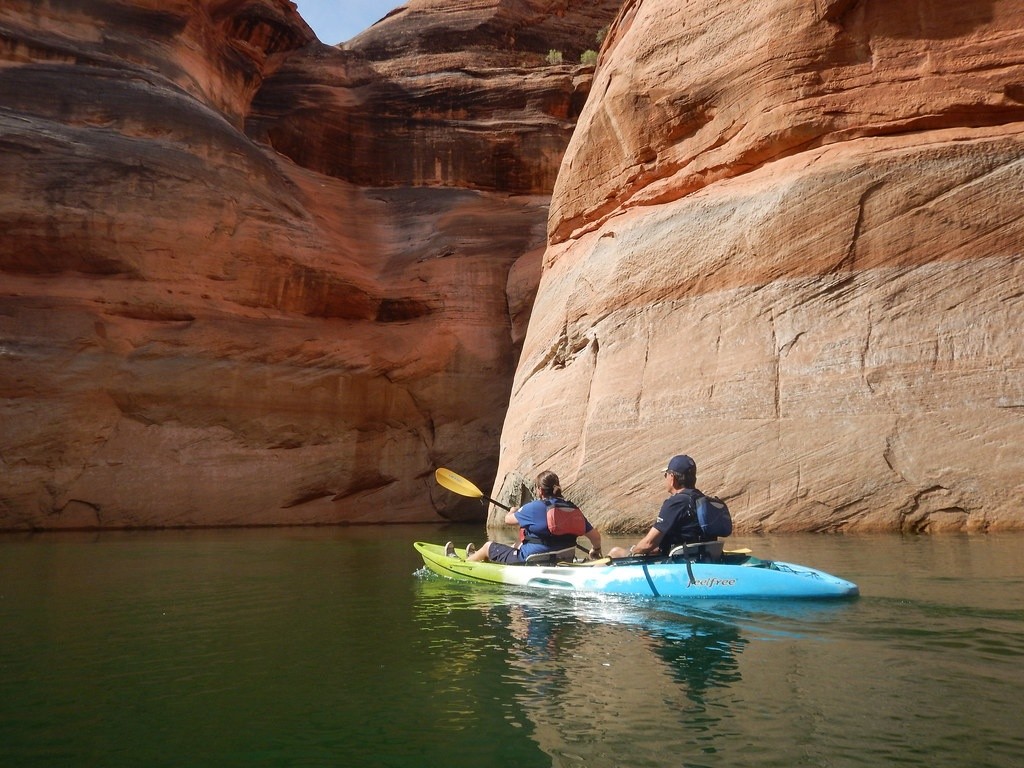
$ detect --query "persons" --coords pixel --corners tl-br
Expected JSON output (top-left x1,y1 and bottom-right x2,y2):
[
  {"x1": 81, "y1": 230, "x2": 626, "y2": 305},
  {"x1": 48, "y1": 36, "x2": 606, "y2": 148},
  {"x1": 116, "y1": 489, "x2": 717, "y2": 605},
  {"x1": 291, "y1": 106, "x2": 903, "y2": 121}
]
[
  {"x1": 445, "y1": 470, "x2": 603, "y2": 565},
  {"x1": 594, "y1": 455, "x2": 732, "y2": 567}
]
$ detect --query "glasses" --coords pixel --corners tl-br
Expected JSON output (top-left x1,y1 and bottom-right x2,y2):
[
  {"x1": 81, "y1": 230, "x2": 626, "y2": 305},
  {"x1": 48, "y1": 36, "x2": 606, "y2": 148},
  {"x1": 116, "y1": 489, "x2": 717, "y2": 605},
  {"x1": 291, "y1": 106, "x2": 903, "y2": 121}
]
[{"x1": 665, "y1": 472, "x2": 672, "y2": 478}]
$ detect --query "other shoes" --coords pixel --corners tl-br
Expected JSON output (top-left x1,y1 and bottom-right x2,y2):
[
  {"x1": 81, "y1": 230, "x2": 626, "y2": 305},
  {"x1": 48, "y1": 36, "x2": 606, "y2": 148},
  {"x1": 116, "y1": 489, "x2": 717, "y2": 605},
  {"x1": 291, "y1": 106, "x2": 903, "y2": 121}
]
[
  {"x1": 445, "y1": 541, "x2": 458, "y2": 558},
  {"x1": 466, "y1": 543, "x2": 475, "y2": 559}
]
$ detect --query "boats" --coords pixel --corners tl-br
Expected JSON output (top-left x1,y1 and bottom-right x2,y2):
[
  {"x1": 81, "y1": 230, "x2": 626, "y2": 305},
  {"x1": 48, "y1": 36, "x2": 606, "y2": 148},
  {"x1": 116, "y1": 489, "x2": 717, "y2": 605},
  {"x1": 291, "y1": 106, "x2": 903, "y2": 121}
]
[{"x1": 413, "y1": 539, "x2": 860, "y2": 603}]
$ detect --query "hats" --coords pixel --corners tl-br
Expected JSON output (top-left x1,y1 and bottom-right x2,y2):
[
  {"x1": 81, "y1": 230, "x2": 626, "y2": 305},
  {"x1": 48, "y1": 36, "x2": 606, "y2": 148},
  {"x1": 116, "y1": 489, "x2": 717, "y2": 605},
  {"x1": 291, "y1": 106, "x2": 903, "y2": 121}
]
[{"x1": 660, "y1": 455, "x2": 696, "y2": 480}]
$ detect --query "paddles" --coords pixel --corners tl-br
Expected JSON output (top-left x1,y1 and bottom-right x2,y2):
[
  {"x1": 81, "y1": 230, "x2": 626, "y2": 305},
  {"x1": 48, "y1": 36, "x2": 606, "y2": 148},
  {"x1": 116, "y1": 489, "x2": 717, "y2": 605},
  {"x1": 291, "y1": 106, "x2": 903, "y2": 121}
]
[
  {"x1": 434, "y1": 467, "x2": 600, "y2": 559},
  {"x1": 557, "y1": 547, "x2": 754, "y2": 567}
]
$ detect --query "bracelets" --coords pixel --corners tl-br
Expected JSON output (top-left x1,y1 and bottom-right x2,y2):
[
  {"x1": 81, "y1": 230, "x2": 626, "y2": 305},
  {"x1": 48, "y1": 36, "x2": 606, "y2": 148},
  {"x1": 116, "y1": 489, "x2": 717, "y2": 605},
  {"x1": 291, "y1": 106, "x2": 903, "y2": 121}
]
[
  {"x1": 630, "y1": 545, "x2": 637, "y2": 556},
  {"x1": 592, "y1": 547, "x2": 601, "y2": 551}
]
